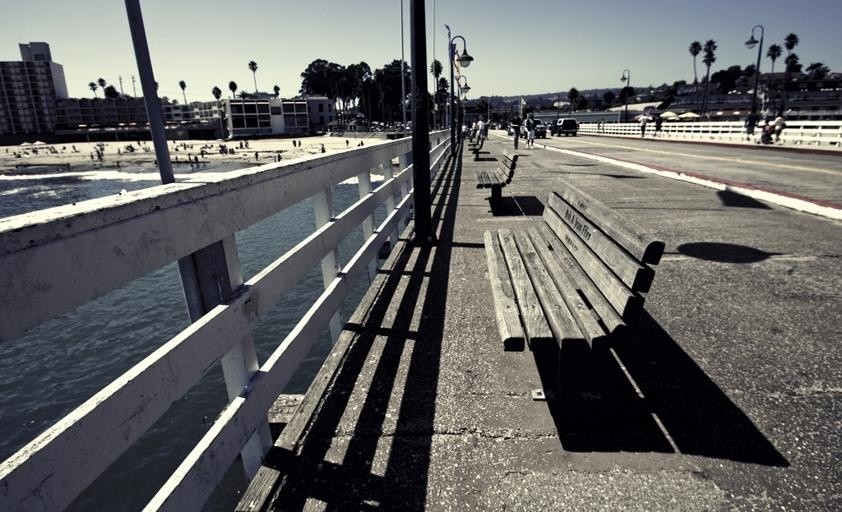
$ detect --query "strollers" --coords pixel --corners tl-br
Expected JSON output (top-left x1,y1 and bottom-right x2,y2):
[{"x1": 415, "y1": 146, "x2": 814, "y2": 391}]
[{"x1": 757, "y1": 127, "x2": 777, "y2": 144}]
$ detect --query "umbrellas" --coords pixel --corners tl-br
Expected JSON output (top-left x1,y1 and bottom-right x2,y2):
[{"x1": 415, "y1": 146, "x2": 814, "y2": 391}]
[
  {"x1": 667, "y1": 116, "x2": 680, "y2": 121},
  {"x1": 678, "y1": 111, "x2": 700, "y2": 118},
  {"x1": 659, "y1": 110, "x2": 677, "y2": 118}
]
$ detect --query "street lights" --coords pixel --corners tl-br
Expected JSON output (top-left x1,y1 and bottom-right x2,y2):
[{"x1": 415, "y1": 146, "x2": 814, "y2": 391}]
[
  {"x1": 454, "y1": 71, "x2": 473, "y2": 147},
  {"x1": 621, "y1": 68, "x2": 630, "y2": 123},
  {"x1": 442, "y1": 21, "x2": 475, "y2": 158},
  {"x1": 744, "y1": 24, "x2": 767, "y2": 136}
]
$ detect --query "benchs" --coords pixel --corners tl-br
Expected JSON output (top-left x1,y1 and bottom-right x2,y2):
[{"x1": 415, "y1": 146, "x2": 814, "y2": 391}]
[
  {"x1": 474, "y1": 148, "x2": 518, "y2": 214},
  {"x1": 466, "y1": 131, "x2": 484, "y2": 160},
  {"x1": 481, "y1": 175, "x2": 665, "y2": 407}
]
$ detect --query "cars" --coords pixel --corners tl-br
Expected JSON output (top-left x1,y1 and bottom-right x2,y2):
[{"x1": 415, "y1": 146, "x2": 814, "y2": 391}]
[
  {"x1": 506, "y1": 116, "x2": 581, "y2": 140},
  {"x1": 371, "y1": 120, "x2": 412, "y2": 132}
]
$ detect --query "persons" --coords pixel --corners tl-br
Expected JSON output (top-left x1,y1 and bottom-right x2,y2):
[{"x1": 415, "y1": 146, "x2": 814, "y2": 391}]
[
  {"x1": 6, "y1": 139, "x2": 365, "y2": 169},
  {"x1": 743, "y1": 108, "x2": 785, "y2": 145},
  {"x1": 461, "y1": 112, "x2": 537, "y2": 150},
  {"x1": 638, "y1": 113, "x2": 650, "y2": 137},
  {"x1": 653, "y1": 115, "x2": 664, "y2": 136}
]
